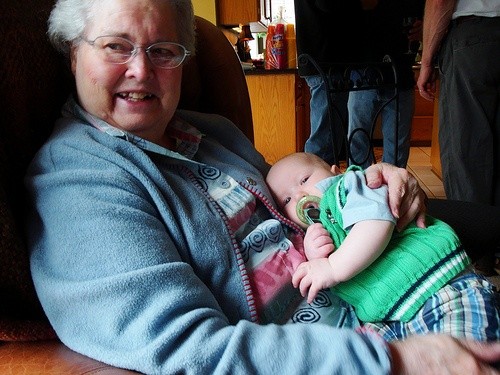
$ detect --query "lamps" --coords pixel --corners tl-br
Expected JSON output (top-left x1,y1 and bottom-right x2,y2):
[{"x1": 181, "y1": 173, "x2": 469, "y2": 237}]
[{"x1": 239, "y1": 25, "x2": 255, "y2": 63}]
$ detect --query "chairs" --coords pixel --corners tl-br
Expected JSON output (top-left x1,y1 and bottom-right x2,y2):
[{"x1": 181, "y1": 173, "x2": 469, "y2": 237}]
[
  {"x1": 295, "y1": 52, "x2": 500, "y2": 276},
  {"x1": 0, "y1": 0, "x2": 254, "y2": 375}
]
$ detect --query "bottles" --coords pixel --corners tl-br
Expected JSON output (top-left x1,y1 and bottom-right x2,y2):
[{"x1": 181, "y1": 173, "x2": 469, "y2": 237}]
[{"x1": 265, "y1": 23, "x2": 296, "y2": 70}]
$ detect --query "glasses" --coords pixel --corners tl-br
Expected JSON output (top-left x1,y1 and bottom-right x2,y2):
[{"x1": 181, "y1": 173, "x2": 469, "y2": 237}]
[{"x1": 79, "y1": 35, "x2": 192, "y2": 70}]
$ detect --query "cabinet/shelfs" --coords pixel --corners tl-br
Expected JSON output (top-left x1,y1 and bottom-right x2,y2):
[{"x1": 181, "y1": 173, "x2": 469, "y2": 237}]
[
  {"x1": 245, "y1": 75, "x2": 306, "y2": 165},
  {"x1": 217, "y1": 0, "x2": 273, "y2": 33}
]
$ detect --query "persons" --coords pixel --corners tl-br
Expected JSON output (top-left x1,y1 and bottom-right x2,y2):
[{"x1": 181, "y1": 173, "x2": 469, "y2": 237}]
[
  {"x1": 266, "y1": 152, "x2": 500, "y2": 343},
  {"x1": 294, "y1": 0, "x2": 415, "y2": 170},
  {"x1": 417, "y1": 0, "x2": 500, "y2": 202},
  {"x1": 21, "y1": 0, "x2": 500, "y2": 375},
  {"x1": 237, "y1": 25, "x2": 254, "y2": 62}
]
